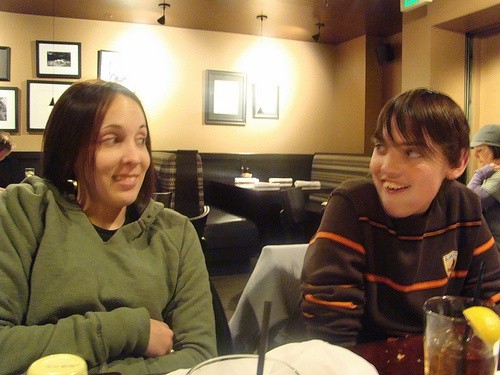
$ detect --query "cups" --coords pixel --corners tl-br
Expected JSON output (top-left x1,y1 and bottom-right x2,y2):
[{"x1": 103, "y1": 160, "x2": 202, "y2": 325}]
[
  {"x1": 24, "y1": 167, "x2": 35, "y2": 177},
  {"x1": 423, "y1": 295, "x2": 499, "y2": 375},
  {"x1": 186, "y1": 354, "x2": 298, "y2": 374}
]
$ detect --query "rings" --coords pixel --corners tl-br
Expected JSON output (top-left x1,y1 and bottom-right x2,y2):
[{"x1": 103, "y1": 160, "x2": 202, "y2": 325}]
[{"x1": 168, "y1": 349, "x2": 174, "y2": 353}]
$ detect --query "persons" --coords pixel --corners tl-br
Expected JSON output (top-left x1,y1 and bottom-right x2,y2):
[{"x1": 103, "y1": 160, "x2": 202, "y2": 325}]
[
  {"x1": 0, "y1": 130, "x2": 25, "y2": 192},
  {"x1": 0, "y1": 79, "x2": 218, "y2": 375},
  {"x1": 468, "y1": 124, "x2": 500, "y2": 252},
  {"x1": 299, "y1": 87, "x2": 500, "y2": 348}
]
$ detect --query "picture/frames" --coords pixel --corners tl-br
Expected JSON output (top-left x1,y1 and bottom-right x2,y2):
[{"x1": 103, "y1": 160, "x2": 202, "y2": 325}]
[
  {"x1": 27, "y1": 80, "x2": 74, "y2": 132},
  {"x1": 0, "y1": 87, "x2": 18, "y2": 132},
  {"x1": 36, "y1": 40, "x2": 81, "y2": 79},
  {"x1": 98, "y1": 50, "x2": 121, "y2": 84},
  {"x1": 252, "y1": 84, "x2": 279, "y2": 119},
  {"x1": 0, "y1": 46, "x2": 11, "y2": 81},
  {"x1": 204, "y1": 69, "x2": 247, "y2": 126}
]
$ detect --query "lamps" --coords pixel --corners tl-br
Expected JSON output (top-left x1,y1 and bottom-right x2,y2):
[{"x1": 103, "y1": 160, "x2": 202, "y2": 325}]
[
  {"x1": 157, "y1": 3, "x2": 171, "y2": 25},
  {"x1": 312, "y1": 23, "x2": 325, "y2": 42}
]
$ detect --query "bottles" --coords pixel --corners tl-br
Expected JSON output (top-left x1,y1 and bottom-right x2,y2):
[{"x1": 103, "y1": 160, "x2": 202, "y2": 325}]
[{"x1": 27, "y1": 353, "x2": 87, "y2": 375}]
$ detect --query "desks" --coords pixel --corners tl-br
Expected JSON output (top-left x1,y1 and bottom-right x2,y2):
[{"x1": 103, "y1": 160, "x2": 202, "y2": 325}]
[{"x1": 209, "y1": 179, "x2": 332, "y2": 239}]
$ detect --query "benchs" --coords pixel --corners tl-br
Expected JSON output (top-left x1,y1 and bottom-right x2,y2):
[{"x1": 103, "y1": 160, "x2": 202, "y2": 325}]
[
  {"x1": 151, "y1": 150, "x2": 258, "y2": 276},
  {"x1": 305, "y1": 152, "x2": 372, "y2": 216}
]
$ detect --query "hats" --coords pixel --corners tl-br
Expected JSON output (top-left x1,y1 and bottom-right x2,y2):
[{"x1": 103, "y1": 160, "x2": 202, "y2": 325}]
[{"x1": 468, "y1": 124, "x2": 500, "y2": 149}]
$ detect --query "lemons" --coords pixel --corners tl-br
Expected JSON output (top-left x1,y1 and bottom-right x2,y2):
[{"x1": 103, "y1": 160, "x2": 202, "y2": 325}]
[{"x1": 463, "y1": 306, "x2": 500, "y2": 349}]
